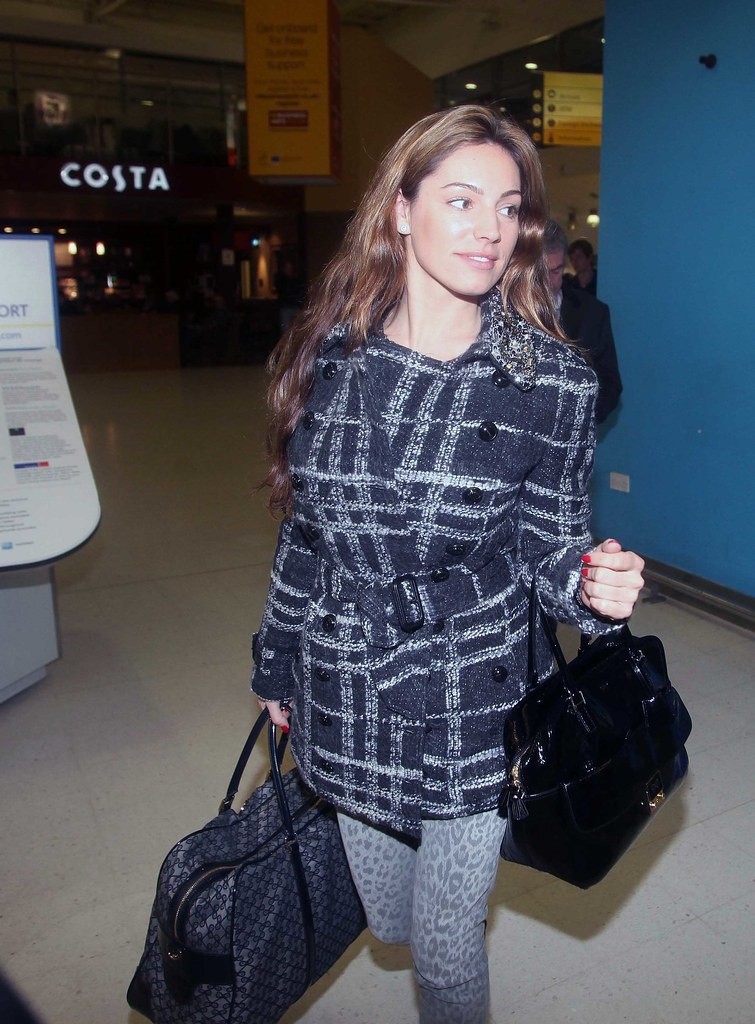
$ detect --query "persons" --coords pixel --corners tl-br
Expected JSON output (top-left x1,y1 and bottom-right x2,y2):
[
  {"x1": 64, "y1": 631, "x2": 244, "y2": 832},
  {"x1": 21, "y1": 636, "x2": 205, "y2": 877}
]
[
  {"x1": 248, "y1": 103, "x2": 646, "y2": 1024},
  {"x1": 541, "y1": 221, "x2": 624, "y2": 634},
  {"x1": 160, "y1": 285, "x2": 230, "y2": 367}
]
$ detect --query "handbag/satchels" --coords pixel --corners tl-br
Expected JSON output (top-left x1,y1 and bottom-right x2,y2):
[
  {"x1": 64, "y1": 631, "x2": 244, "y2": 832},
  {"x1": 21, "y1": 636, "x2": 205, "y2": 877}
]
[
  {"x1": 497, "y1": 546, "x2": 692, "y2": 889},
  {"x1": 127, "y1": 705, "x2": 368, "y2": 1024}
]
